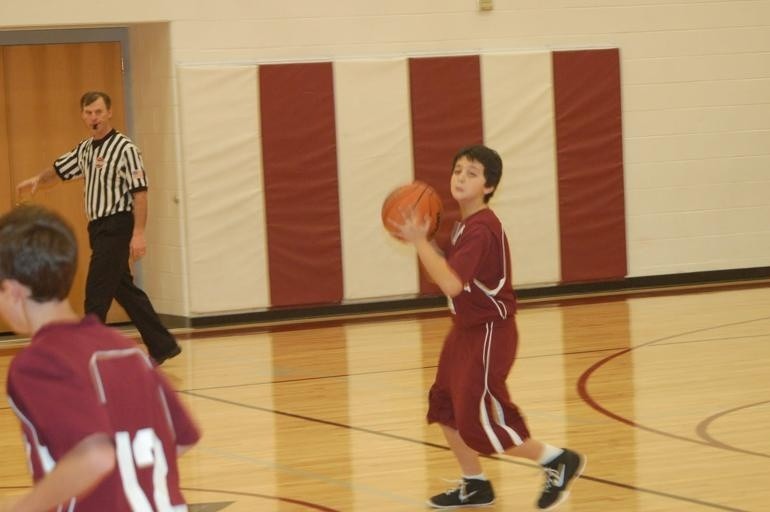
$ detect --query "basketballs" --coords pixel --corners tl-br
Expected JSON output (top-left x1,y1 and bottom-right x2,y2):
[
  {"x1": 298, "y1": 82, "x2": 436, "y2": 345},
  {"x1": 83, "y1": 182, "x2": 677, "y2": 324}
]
[{"x1": 382, "y1": 183, "x2": 442, "y2": 242}]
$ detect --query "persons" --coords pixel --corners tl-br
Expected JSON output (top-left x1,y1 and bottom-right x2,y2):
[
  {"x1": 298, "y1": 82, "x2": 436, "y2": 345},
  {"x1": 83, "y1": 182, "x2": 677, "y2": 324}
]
[
  {"x1": 385, "y1": 144, "x2": 587, "y2": 511},
  {"x1": 14, "y1": 90, "x2": 183, "y2": 370},
  {"x1": 0, "y1": 199, "x2": 202, "y2": 512}
]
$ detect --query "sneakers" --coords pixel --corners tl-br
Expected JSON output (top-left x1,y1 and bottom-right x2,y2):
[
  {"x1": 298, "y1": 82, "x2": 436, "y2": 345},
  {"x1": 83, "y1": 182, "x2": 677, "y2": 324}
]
[
  {"x1": 429, "y1": 477, "x2": 495, "y2": 508},
  {"x1": 538, "y1": 448, "x2": 587, "y2": 511}
]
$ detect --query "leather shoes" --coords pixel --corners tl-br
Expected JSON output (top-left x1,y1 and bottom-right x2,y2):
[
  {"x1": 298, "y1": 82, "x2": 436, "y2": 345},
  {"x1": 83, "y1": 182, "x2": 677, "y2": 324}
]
[{"x1": 148, "y1": 342, "x2": 181, "y2": 367}]
